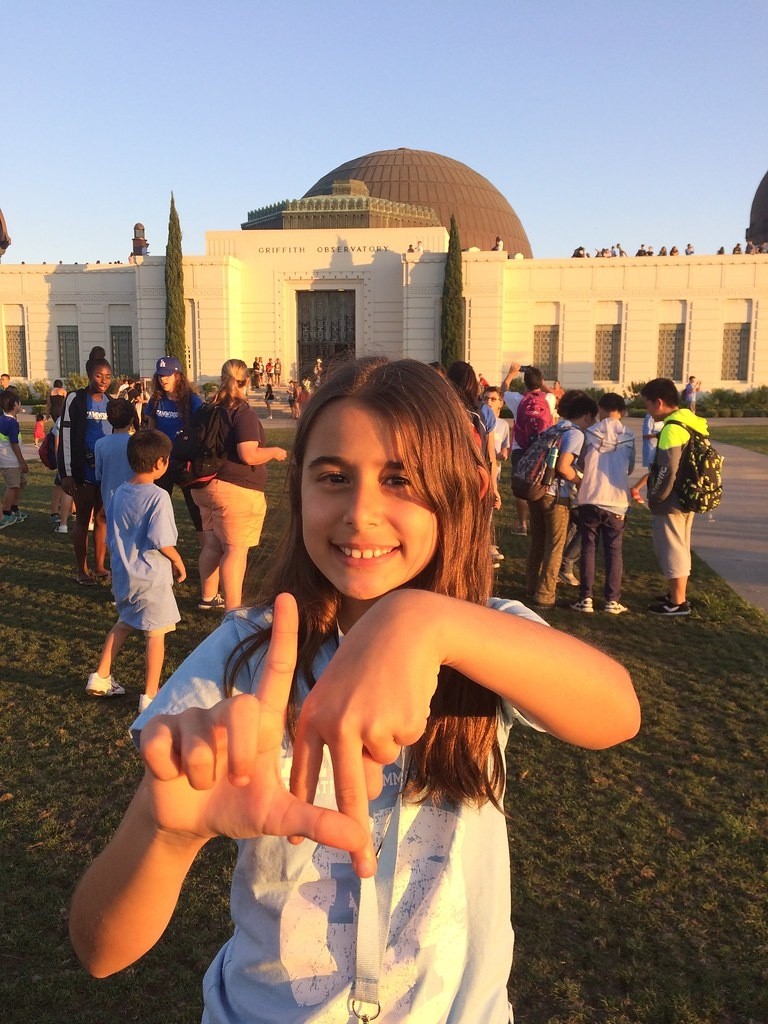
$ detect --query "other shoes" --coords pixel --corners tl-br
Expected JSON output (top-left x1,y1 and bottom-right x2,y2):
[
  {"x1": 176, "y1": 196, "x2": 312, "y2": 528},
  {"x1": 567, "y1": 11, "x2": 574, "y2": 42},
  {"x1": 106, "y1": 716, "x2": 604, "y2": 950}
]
[
  {"x1": 510, "y1": 526, "x2": 529, "y2": 535},
  {"x1": 630, "y1": 487, "x2": 645, "y2": 503},
  {"x1": 492, "y1": 546, "x2": 504, "y2": 559},
  {"x1": 558, "y1": 571, "x2": 579, "y2": 585}
]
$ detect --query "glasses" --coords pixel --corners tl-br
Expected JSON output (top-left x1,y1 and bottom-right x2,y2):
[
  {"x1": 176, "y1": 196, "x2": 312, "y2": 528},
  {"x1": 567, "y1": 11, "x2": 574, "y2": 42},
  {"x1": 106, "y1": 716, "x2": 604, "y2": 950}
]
[{"x1": 483, "y1": 396, "x2": 500, "y2": 402}]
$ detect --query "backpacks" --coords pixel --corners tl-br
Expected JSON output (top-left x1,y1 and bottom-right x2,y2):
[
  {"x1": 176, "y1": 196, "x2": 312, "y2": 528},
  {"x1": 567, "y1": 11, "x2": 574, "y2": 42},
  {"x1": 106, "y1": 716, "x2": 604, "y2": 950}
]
[
  {"x1": 511, "y1": 424, "x2": 581, "y2": 502},
  {"x1": 171, "y1": 402, "x2": 229, "y2": 488},
  {"x1": 512, "y1": 390, "x2": 553, "y2": 451},
  {"x1": 39, "y1": 427, "x2": 58, "y2": 470},
  {"x1": 682, "y1": 384, "x2": 690, "y2": 401},
  {"x1": 665, "y1": 420, "x2": 724, "y2": 514}
]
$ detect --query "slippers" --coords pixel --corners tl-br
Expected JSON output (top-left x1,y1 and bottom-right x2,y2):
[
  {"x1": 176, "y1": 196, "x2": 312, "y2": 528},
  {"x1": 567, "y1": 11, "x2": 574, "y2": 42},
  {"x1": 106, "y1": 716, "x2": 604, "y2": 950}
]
[{"x1": 75, "y1": 576, "x2": 99, "y2": 588}]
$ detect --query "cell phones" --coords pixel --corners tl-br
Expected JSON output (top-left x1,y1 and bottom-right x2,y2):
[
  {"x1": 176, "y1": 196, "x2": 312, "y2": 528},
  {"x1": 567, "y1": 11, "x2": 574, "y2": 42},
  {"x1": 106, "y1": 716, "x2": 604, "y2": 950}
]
[{"x1": 520, "y1": 366, "x2": 530, "y2": 372}]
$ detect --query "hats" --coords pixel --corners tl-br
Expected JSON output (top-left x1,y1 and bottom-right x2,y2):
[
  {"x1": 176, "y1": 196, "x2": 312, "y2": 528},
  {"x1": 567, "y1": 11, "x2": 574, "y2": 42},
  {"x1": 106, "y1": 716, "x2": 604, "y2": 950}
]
[
  {"x1": 317, "y1": 359, "x2": 322, "y2": 362},
  {"x1": 155, "y1": 355, "x2": 182, "y2": 375}
]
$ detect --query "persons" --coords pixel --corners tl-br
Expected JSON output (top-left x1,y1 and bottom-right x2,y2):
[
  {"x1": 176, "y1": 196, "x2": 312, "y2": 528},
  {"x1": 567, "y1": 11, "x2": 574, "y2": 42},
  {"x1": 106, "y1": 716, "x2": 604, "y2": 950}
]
[
  {"x1": 571, "y1": 393, "x2": 636, "y2": 614},
  {"x1": 571, "y1": 239, "x2": 766, "y2": 258},
  {"x1": 630, "y1": 413, "x2": 665, "y2": 504},
  {"x1": 685, "y1": 376, "x2": 701, "y2": 414},
  {"x1": 57, "y1": 345, "x2": 120, "y2": 585},
  {"x1": 22, "y1": 261, "x2": 123, "y2": 264},
  {"x1": 408, "y1": 241, "x2": 423, "y2": 251},
  {"x1": 116, "y1": 376, "x2": 151, "y2": 433},
  {"x1": 189, "y1": 359, "x2": 287, "y2": 615},
  {"x1": 429, "y1": 359, "x2": 599, "y2": 608},
  {"x1": 70, "y1": 355, "x2": 642, "y2": 1024},
  {"x1": 0, "y1": 373, "x2": 94, "y2": 533},
  {"x1": 492, "y1": 237, "x2": 503, "y2": 251},
  {"x1": 641, "y1": 378, "x2": 710, "y2": 615},
  {"x1": 244, "y1": 357, "x2": 323, "y2": 421},
  {"x1": 86, "y1": 429, "x2": 187, "y2": 713},
  {"x1": 144, "y1": 356, "x2": 204, "y2": 545}
]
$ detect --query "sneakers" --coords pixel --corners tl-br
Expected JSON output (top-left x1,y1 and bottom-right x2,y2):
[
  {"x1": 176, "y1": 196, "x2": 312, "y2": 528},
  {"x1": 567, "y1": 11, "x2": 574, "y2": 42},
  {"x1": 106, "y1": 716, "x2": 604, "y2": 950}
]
[
  {"x1": 197, "y1": 594, "x2": 225, "y2": 610},
  {"x1": 0, "y1": 515, "x2": 17, "y2": 529},
  {"x1": 13, "y1": 510, "x2": 30, "y2": 518},
  {"x1": 571, "y1": 598, "x2": 594, "y2": 612},
  {"x1": 655, "y1": 593, "x2": 670, "y2": 603},
  {"x1": 605, "y1": 601, "x2": 628, "y2": 614},
  {"x1": 54, "y1": 523, "x2": 68, "y2": 533},
  {"x1": 85, "y1": 673, "x2": 125, "y2": 696},
  {"x1": 648, "y1": 601, "x2": 691, "y2": 615},
  {"x1": 138, "y1": 694, "x2": 153, "y2": 713},
  {"x1": 50, "y1": 514, "x2": 61, "y2": 523}
]
